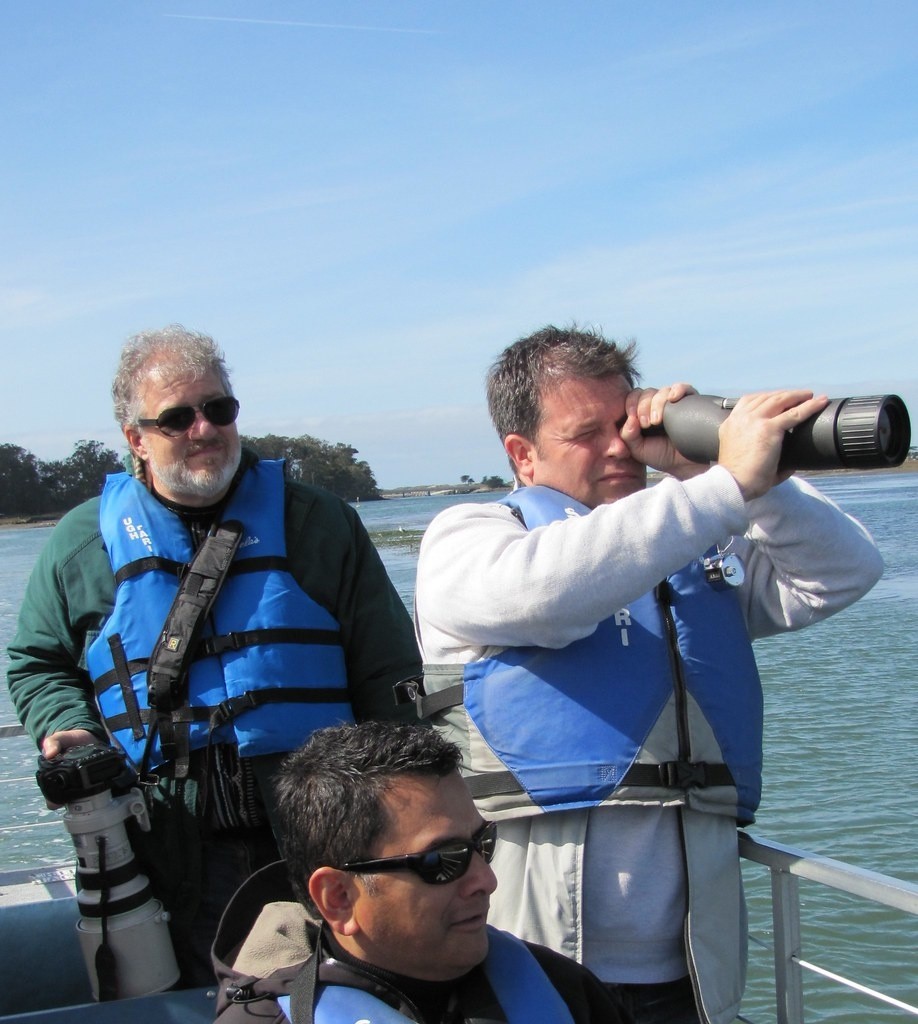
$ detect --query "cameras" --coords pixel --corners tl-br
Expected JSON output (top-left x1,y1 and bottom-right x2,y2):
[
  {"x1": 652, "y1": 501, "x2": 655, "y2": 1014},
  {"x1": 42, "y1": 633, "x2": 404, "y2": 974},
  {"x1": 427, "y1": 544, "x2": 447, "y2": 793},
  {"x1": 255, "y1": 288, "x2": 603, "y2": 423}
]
[{"x1": 34, "y1": 742, "x2": 181, "y2": 1002}]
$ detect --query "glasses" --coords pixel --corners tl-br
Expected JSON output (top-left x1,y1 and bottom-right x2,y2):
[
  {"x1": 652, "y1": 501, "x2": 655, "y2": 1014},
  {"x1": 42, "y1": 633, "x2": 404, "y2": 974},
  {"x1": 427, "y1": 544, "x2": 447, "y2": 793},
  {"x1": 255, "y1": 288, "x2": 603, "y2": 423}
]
[
  {"x1": 138, "y1": 395, "x2": 240, "y2": 437},
  {"x1": 343, "y1": 820, "x2": 498, "y2": 885}
]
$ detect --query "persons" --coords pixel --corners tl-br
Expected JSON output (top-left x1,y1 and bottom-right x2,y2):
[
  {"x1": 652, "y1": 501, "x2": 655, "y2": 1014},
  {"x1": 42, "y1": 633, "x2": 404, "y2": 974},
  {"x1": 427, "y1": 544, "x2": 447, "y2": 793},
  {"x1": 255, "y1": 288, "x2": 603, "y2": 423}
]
[
  {"x1": 415, "y1": 326, "x2": 886, "y2": 1024},
  {"x1": 216, "y1": 721, "x2": 633, "y2": 1024},
  {"x1": 6, "y1": 326, "x2": 424, "y2": 987}
]
